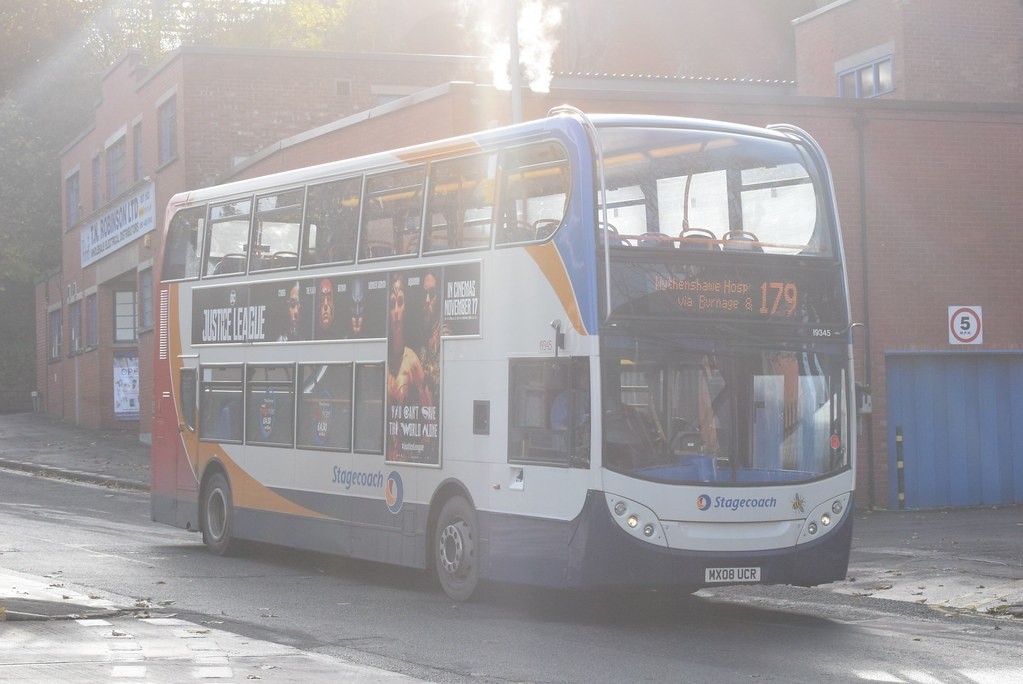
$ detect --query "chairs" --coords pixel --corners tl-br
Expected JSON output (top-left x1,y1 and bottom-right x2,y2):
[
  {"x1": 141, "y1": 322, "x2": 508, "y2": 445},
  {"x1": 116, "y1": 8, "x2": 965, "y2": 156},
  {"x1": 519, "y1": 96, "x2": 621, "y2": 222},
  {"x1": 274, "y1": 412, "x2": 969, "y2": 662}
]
[{"x1": 211, "y1": 218, "x2": 764, "y2": 279}]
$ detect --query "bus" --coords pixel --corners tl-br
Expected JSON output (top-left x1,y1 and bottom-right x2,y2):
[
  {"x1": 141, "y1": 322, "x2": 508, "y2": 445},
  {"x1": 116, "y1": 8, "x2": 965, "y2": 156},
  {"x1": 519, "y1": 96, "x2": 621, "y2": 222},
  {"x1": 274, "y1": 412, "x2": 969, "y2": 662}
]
[{"x1": 149, "y1": 104, "x2": 865, "y2": 611}]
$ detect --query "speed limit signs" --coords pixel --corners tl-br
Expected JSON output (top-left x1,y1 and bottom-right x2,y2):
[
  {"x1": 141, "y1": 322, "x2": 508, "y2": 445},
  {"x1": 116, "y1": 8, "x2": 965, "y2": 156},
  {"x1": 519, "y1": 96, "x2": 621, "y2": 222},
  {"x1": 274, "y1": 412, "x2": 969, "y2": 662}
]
[{"x1": 949, "y1": 305, "x2": 982, "y2": 343}]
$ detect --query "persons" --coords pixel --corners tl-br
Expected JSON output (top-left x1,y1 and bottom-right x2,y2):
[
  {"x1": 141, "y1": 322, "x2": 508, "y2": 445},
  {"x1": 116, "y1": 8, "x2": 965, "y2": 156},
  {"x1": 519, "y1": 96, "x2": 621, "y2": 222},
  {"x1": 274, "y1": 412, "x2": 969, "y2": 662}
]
[
  {"x1": 278, "y1": 287, "x2": 301, "y2": 341},
  {"x1": 389, "y1": 277, "x2": 434, "y2": 461},
  {"x1": 320, "y1": 279, "x2": 334, "y2": 332},
  {"x1": 551, "y1": 380, "x2": 635, "y2": 429},
  {"x1": 417, "y1": 274, "x2": 450, "y2": 386},
  {"x1": 350, "y1": 278, "x2": 365, "y2": 334}
]
